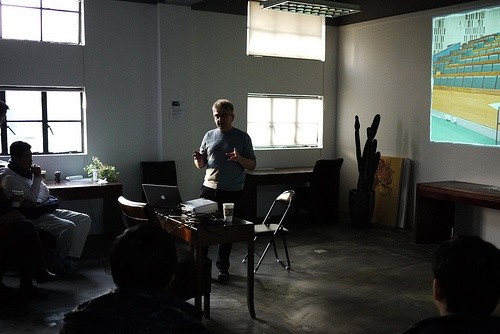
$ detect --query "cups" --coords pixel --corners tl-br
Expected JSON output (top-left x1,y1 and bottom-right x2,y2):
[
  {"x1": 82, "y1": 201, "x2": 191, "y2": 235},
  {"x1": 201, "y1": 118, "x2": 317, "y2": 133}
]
[{"x1": 222, "y1": 203, "x2": 234, "y2": 223}]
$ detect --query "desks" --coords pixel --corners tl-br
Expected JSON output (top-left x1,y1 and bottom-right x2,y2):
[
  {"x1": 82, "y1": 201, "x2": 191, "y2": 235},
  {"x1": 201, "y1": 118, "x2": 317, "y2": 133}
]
[
  {"x1": 245, "y1": 166, "x2": 313, "y2": 221},
  {"x1": 38, "y1": 179, "x2": 125, "y2": 251},
  {"x1": 416, "y1": 181, "x2": 500, "y2": 245},
  {"x1": 153, "y1": 207, "x2": 256, "y2": 320}
]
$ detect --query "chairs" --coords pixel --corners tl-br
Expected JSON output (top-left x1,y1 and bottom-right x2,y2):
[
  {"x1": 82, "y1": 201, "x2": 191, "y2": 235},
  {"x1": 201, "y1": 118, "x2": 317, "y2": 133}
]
[
  {"x1": 433, "y1": 32, "x2": 500, "y2": 94},
  {"x1": 117, "y1": 197, "x2": 213, "y2": 316},
  {"x1": 242, "y1": 190, "x2": 296, "y2": 273},
  {"x1": 300, "y1": 158, "x2": 343, "y2": 226}
]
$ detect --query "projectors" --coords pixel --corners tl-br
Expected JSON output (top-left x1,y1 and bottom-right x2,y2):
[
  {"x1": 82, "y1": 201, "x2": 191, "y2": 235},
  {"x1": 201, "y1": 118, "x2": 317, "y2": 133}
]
[{"x1": 182, "y1": 197, "x2": 219, "y2": 216}]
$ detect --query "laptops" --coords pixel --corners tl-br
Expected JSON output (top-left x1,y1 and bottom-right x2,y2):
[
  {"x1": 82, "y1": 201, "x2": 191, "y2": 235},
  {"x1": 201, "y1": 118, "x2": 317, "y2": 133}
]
[{"x1": 143, "y1": 183, "x2": 184, "y2": 210}]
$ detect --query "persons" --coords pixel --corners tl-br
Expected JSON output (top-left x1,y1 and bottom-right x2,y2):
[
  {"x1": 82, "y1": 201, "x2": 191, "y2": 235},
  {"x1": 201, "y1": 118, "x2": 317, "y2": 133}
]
[
  {"x1": 58, "y1": 223, "x2": 209, "y2": 334},
  {"x1": 402, "y1": 236, "x2": 500, "y2": 334},
  {"x1": 0, "y1": 141, "x2": 92, "y2": 280},
  {"x1": 192, "y1": 99, "x2": 256, "y2": 281}
]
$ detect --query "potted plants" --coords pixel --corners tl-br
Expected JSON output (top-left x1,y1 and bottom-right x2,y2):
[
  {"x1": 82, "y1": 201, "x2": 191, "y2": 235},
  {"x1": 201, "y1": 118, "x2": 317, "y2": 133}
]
[{"x1": 348, "y1": 114, "x2": 384, "y2": 226}]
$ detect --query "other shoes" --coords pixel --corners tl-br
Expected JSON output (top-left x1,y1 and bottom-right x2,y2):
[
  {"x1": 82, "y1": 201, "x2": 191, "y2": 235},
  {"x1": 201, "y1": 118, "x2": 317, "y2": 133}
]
[{"x1": 218, "y1": 269, "x2": 230, "y2": 282}]
